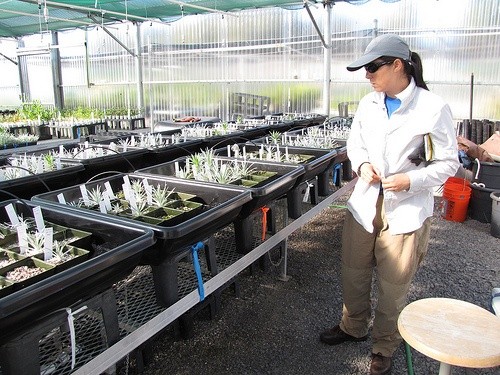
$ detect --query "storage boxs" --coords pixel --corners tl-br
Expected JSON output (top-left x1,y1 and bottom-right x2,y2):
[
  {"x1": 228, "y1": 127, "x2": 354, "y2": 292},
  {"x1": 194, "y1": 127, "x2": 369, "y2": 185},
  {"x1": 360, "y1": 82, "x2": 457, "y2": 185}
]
[
  {"x1": 247, "y1": 132, "x2": 347, "y2": 196},
  {"x1": 214, "y1": 142, "x2": 337, "y2": 220},
  {"x1": 0, "y1": 199, "x2": 153, "y2": 374},
  {"x1": 66, "y1": 146, "x2": 146, "y2": 184},
  {"x1": 133, "y1": 153, "x2": 305, "y2": 252},
  {"x1": 467, "y1": 162, "x2": 500, "y2": 223},
  {"x1": 33, "y1": 174, "x2": 250, "y2": 308},
  {"x1": 0, "y1": 154, "x2": 86, "y2": 202}
]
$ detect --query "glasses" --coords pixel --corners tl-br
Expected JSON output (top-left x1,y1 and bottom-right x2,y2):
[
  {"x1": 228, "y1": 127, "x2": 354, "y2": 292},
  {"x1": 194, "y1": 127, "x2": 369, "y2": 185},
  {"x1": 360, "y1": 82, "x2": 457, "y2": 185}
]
[{"x1": 364, "y1": 60, "x2": 391, "y2": 73}]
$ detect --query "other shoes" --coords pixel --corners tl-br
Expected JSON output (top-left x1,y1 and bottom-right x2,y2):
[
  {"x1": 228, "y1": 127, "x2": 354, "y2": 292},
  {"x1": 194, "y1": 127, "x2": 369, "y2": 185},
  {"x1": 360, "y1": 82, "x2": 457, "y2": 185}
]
[
  {"x1": 370, "y1": 351, "x2": 394, "y2": 375},
  {"x1": 320, "y1": 324, "x2": 369, "y2": 346}
]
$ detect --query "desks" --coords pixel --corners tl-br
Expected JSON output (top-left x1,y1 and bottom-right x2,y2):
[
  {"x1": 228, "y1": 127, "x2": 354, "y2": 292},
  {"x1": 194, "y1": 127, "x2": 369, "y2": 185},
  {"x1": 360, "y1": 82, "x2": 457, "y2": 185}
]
[{"x1": 398, "y1": 298, "x2": 500, "y2": 375}]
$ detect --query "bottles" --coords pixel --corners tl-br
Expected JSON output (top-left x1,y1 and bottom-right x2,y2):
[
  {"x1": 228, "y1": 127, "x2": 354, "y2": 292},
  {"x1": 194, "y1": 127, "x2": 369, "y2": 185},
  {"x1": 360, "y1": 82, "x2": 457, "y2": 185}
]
[{"x1": 491, "y1": 287, "x2": 500, "y2": 318}]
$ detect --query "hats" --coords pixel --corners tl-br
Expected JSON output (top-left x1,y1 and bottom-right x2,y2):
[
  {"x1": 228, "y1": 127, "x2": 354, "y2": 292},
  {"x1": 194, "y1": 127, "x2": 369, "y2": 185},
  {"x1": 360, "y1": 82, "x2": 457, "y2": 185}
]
[{"x1": 346, "y1": 34, "x2": 413, "y2": 72}]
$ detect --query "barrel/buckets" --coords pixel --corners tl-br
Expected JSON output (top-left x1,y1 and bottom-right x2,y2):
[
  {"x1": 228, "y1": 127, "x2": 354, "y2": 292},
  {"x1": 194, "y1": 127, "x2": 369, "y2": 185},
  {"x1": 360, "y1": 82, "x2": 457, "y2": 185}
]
[
  {"x1": 490, "y1": 191, "x2": 500, "y2": 239},
  {"x1": 440, "y1": 159, "x2": 500, "y2": 224}
]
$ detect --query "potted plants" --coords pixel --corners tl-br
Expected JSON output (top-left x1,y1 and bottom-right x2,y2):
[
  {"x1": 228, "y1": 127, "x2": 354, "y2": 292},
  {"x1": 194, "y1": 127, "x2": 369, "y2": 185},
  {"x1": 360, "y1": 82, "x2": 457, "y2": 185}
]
[{"x1": 0, "y1": 100, "x2": 353, "y2": 293}]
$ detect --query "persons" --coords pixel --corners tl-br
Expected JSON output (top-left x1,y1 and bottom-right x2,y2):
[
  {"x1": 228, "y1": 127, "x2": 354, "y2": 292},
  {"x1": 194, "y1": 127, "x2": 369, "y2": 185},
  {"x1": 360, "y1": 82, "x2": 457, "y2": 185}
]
[{"x1": 320, "y1": 34, "x2": 460, "y2": 375}]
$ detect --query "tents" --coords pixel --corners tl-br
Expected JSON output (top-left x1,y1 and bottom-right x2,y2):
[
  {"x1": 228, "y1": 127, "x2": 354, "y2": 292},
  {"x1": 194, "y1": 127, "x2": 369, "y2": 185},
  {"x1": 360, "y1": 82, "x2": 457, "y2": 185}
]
[{"x1": 0, "y1": 0, "x2": 333, "y2": 124}]
